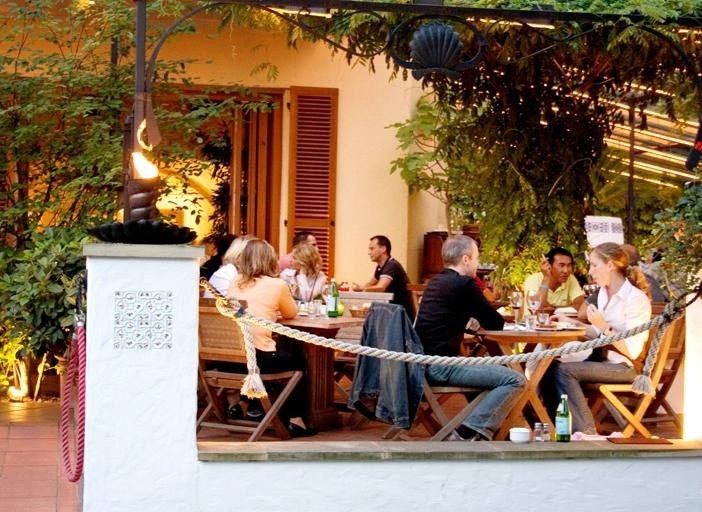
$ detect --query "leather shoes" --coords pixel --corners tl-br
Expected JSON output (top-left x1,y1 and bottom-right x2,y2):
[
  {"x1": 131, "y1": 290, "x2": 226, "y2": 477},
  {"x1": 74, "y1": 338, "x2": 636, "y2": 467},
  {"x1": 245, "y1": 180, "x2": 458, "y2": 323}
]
[
  {"x1": 288, "y1": 421, "x2": 319, "y2": 437},
  {"x1": 246, "y1": 408, "x2": 264, "y2": 421},
  {"x1": 228, "y1": 402, "x2": 243, "y2": 419}
]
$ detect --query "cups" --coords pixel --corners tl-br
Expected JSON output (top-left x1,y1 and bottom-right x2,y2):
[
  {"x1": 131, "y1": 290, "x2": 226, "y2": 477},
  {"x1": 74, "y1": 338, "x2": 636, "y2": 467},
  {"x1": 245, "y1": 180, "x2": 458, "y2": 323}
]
[{"x1": 297, "y1": 300, "x2": 322, "y2": 318}]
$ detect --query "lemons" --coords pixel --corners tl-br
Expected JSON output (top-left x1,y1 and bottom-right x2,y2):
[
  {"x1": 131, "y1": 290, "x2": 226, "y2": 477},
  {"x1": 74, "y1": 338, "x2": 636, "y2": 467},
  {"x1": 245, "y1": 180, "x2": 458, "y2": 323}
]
[{"x1": 337, "y1": 304, "x2": 344, "y2": 315}]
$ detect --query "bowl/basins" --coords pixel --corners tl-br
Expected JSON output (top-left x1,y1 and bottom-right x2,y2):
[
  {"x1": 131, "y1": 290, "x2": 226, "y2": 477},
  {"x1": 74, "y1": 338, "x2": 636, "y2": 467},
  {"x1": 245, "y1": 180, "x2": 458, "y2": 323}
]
[
  {"x1": 350, "y1": 309, "x2": 367, "y2": 318},
  {"x1": 509, "y1": 428, "x2": 530, "y2": 443}
]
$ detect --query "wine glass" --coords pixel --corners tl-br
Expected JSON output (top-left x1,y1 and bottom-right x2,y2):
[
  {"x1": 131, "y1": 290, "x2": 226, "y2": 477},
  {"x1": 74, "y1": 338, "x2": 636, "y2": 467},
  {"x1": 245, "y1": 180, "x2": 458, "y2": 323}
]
[{"x1": 512, "y1": 291, "x2": 548, "y2": 330}]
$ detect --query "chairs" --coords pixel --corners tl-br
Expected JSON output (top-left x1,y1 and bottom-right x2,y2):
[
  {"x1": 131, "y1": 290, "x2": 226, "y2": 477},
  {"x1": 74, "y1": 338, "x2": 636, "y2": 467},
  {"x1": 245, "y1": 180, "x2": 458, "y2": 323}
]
[
  {"x1": 334, "y1": 292, "x2": 394, "y2": 419},
  {"x1": 370, "y1": 300, "x2": 488, "y2": 441},
  {"x1": 198, "y1": 298, "x2": 303, "y2": 441},
  {"x1": 640, "y1": 303, "x2": 684, "y2": 428},
  {"x1": 584, "y1": 318, "x2": 676, "y2": 441}
]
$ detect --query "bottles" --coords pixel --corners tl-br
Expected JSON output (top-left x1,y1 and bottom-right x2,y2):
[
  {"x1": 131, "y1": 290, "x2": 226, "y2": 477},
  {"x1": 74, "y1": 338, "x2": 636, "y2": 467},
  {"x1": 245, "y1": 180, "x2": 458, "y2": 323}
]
[
  {"x1": 543, "y1": 421, "x2": 550, "y2": 442},
  {"x1": 555, "y1": 393, "x2": 575, "y2": 441},
  {"x1": 328, "y1": 278, "x2": 338, "y2": 317},
  {"x1": 535, "y1": 422, "x2": 541, "y2": 441}
]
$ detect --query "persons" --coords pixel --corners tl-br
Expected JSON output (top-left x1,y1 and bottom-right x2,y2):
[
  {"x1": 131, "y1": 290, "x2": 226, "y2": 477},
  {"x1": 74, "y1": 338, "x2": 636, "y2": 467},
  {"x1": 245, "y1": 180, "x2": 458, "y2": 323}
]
[
  {"x1": 226, "y1": 238, "x2": 318, "y2": 437},
  {"x1": 204, "y1": 234, "x2": 259, "y2": 423},
  {"x1": 279, "y1": 231, "x2": 319, "y2": 273},
  {"x1": 279, "y1": 244, "x2": 328, "y2": 302},
  {"x1": 512, "y1": 246, "x2": 587, "y2": 354},
  {"x1": 538, "y1": 243, "x2": 665, "y2": 435},
  {"x1": 200, "y1": 235, "x2": 239, "y2": 279},
  {"x1": 351, "y1": 235, "x2": 416, "y2": 327},
  {"x1": 417, "y1": 232, "x2": 528, "y2": 442}
]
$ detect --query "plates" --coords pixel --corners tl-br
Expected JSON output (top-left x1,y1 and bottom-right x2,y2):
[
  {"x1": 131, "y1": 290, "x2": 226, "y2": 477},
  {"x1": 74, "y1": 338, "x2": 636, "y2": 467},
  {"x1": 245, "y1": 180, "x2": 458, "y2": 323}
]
[{"x1": 536, "y1": 322, "x2": 586, "y2": 331}]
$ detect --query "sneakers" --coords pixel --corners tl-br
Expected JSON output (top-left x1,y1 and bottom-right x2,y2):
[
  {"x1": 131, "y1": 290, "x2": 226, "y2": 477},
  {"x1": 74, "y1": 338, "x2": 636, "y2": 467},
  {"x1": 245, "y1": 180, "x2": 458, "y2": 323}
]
[{"x1": 447, "y1": 427, "x2": 480, "y2": 441}]
